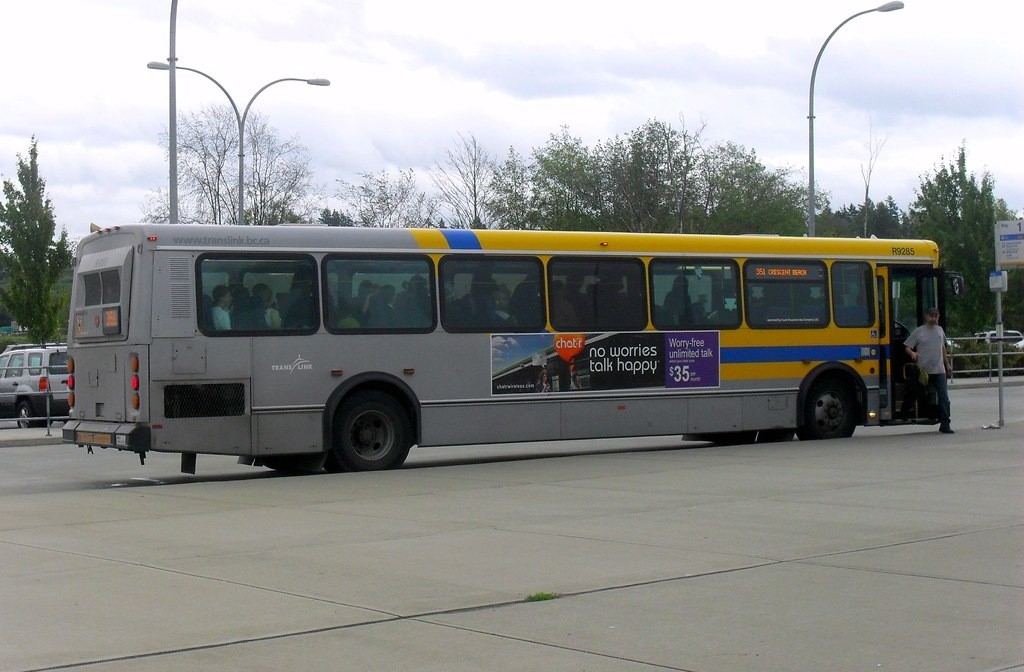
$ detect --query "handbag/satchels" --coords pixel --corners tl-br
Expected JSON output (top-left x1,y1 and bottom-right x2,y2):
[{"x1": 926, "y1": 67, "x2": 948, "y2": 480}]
[{"x1": 917, "y1": 363, "x2": 928, "y2": 385}]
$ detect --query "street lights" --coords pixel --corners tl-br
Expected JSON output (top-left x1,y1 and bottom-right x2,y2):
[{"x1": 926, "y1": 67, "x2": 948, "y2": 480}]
[
  {"x1": 807, "y1": 1, "x2": 905, "y2": 233},
  {"x1": 146, "y1": 60, "x2": 331, "y2": 225}
]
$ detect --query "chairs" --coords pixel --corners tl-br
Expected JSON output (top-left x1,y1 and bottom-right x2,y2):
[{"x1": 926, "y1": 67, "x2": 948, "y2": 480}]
[
  {"x1": 655, "y1": 315, "x2": 679, "y2": 326},
  {"x1": 202, "y1": 294, "x2": 215, "y2": 330}
]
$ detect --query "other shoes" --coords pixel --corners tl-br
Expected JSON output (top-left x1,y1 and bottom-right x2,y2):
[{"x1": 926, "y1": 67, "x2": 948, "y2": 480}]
[
  {"x1": 901, "y1": 404, "x2": 909, "y2": 422},
  {"x1": 940, "y1": 426, "x2": 954, "y2": 433}
]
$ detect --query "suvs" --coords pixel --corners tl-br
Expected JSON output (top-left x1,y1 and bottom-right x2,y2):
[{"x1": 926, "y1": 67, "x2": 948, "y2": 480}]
[{"x1": 1, "y1": 342, "x2": 71, "y2": 428}]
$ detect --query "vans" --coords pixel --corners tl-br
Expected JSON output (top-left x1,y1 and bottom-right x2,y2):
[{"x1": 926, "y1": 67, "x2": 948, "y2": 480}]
[{"x1": 974, "y1": 331, "x2": 1023, "y2": 346}]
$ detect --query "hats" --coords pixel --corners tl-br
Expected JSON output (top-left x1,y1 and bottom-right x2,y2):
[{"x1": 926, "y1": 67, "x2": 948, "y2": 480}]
[{"x1": 927, "y1": 308, "x2": 939, "y2": 314}]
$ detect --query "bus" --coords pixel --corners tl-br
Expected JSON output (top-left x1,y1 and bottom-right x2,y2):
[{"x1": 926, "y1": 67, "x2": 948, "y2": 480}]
[{"x1": 63, "y1": 218, "x2": 969, "y2": 474}]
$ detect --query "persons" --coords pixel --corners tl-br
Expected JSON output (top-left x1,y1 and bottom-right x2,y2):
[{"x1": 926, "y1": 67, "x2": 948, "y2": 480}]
[
  {"x1": 536, "y1": 369, "x2": 552, "y2": 393},
  {"x1": 211, "y1": 270, "x2": 821, "y2": 333},
  {"x1": 570, "y1": 372, "x2": 583, "y2": 390},
  {"x1": 901, "y1": 307, "x2": 955, "y2": 434}
]
